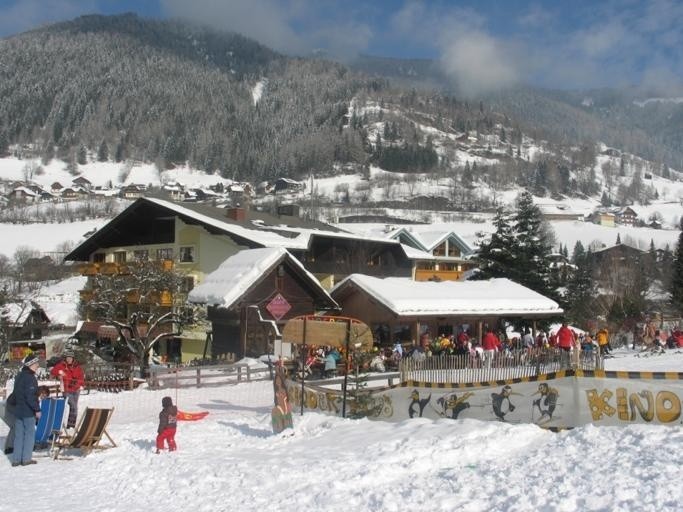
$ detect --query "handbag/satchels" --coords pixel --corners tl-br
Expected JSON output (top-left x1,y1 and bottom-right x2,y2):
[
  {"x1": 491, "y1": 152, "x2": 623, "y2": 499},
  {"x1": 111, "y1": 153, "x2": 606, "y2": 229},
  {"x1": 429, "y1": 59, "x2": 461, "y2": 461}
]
[{"x1": 6, "y1": 390, "x2": 15, "y2": 414}]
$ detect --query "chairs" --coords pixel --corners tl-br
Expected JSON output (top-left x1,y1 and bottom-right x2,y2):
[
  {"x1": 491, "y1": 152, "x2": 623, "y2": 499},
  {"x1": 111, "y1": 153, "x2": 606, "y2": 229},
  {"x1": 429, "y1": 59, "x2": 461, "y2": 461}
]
[{"x1": 32, "y1": 397, "x2": 116, "y2": 460}]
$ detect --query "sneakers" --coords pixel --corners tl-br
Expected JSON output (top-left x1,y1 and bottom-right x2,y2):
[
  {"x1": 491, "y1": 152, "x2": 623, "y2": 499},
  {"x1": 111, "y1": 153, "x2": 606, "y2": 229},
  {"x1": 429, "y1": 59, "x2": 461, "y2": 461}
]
[
  {"x1": 11, "y1": 460, "x2": 37, "y2": 466},
  {"x1": 5, "y1": 447, "x2": 13, "y2": 454}
]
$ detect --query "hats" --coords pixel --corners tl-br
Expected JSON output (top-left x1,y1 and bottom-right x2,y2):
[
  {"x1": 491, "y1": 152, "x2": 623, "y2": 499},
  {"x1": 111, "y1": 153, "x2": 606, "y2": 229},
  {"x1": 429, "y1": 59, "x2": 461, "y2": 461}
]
[
  {"x1": 63, "y1": 348, "x2": 76, "y2": 358},
  {"x1": 24, "y1": 353, "x2": 40, "y2": 367}
]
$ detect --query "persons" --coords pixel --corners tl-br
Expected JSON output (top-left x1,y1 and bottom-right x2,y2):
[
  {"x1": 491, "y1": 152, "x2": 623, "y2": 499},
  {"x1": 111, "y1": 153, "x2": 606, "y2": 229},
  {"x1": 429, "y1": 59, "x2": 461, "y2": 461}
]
[
  {"x1": 10, "y1": 353, "x2": 40, "y2": 466},
  {"x1": 50, "y1": 350, "x2": 84, "y2": 430},
  {"x1": 154, "y1": 395, "x2": 177, "y2": 454},
  {"x1": 630, "y1": 319, "x2": 683, "y2": 350},
  {"x1": 317, "y1": 351, "x2": 337, "y2": 379},
  {"x1": 3, "y1": 384, "x2": 49, "y2": 453},
  {"x1": 379, "y1": 322, "x2": 612, "y2": 371}
]
